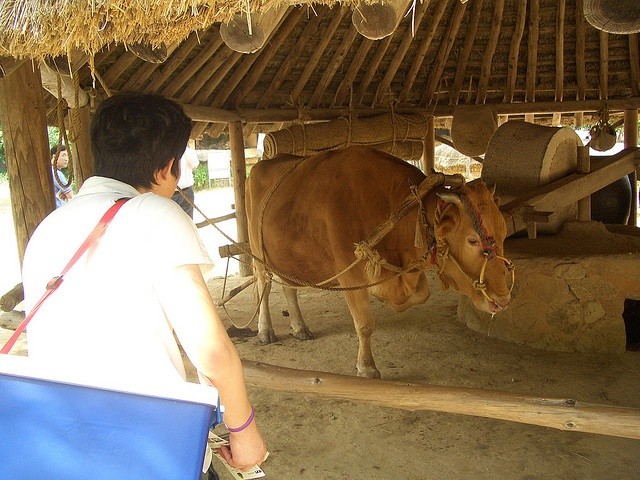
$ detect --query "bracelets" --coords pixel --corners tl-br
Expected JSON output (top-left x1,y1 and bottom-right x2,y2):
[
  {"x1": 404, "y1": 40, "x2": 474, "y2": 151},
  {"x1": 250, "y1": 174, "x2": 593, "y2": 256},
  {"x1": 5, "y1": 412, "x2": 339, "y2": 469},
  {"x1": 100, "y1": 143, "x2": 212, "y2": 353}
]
[{"x1": 220, "y1": 409, "x2": 256, "y2": 431}]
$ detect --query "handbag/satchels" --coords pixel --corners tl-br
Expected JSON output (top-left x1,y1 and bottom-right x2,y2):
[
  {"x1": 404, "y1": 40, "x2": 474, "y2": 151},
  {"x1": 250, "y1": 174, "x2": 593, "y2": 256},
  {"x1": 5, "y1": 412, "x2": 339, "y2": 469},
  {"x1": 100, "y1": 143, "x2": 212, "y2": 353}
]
[{"x1": 0, "y1": 197, "x2": 223, "y2": 478}]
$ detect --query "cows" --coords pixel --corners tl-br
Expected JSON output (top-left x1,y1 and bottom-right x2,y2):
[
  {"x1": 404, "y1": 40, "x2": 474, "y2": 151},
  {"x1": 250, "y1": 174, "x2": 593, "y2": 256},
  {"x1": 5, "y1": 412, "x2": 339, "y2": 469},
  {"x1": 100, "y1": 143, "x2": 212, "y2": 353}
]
[{"x1": 244, "y1": 146, "x2": 514, "y2": 378}]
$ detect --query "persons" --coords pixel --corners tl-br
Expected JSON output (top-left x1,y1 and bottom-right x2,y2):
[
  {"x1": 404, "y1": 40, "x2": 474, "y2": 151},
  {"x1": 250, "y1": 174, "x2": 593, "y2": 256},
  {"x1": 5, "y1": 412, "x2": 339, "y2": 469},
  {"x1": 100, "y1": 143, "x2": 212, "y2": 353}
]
[
  {"x1": 49, "y1": 144, "x2": 73, "y2": 210},
  {"x1": 23, "y1": 94, "x2": 267, "y2": 478},
  {"x1": 174, "y1": 145, "x2": 199, "y2": 221}
]
